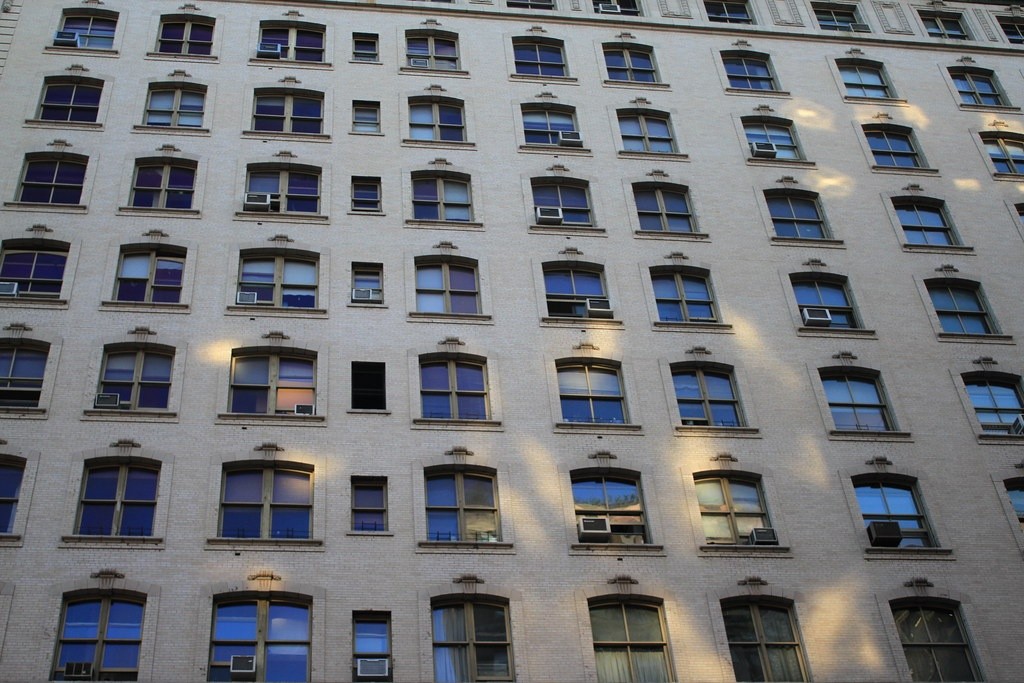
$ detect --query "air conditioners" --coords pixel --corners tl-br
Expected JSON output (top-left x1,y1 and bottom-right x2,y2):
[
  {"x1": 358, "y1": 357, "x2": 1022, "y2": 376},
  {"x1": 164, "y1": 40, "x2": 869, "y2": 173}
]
[
  {"x1": 599, "y1": 4, "x2": 620, "y2": 14},
  {"x1": 256, "y1": 42, "x2": 282, "y2": 57},
  {"x1": 0, "y1": 282, "x2": 20, "y2": 297},
  {"x1": 586, "y1": 298, "x2": 614, "y2": 316},
  {"x1": 848, "y1": 23, "x2": 871, "y2": 32},
  {"x1": 535, "y1": 131, "x2": 584, "y2": 226},
  {"x1": 295, "y1": 404, "x2": 316, "y2": 415},
  {"x1": 230, "y1": 655, "x2": 257, "y2": 680},
  {"x1": 752, "y1": 142, "x2": 777, "y2": 157},
  {"x1": 357, "y1": 659, "x2": 389, "y2": 679},
  {"x1": 750, "y1": 527, "x2": 778, "y2": 545},
  {"x1": 577, "y1": 516, "x2": 613, "y2": 543},
  {"x1": 54, "y1": 30, "x2": 82, "y2": 47},
  {"x1": 237, "y1": 193, "x2": 373, "y2": 306},
  {"x1": 411, "y1": 59, "x2": 429, "y2": 67},
  {"x1": 95, "y1": 392, "x2": 120, "y2": 408},
  {"x1": 802, "y1": 308, "x2": 831, "y2": 326},
  {"x1": 64, "y1": 661, "x2": 94, "y2": 681},
  {"x1": 866, "y1": 521, "x2": 904, "y2": 547},
  {"x1": 1012, "y1": 414, "x2": 1024, "y2": 435}
]
